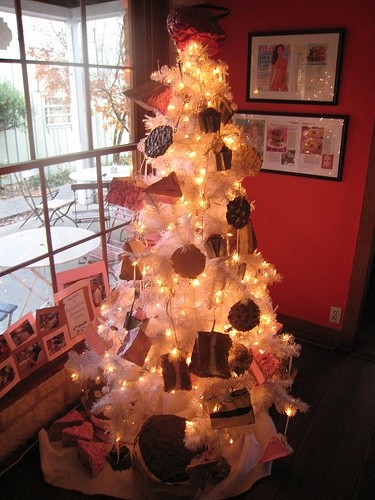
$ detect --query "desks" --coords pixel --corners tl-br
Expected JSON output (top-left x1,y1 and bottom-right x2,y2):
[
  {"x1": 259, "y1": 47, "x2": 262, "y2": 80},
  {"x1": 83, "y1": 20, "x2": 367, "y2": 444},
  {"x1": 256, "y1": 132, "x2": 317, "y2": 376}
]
[
  {"x1": 0, "y1": 227, "x2": 101, "y2": 320},
  {"x1": 68, "y1": 166, "x2": 130, "y2": 229}
]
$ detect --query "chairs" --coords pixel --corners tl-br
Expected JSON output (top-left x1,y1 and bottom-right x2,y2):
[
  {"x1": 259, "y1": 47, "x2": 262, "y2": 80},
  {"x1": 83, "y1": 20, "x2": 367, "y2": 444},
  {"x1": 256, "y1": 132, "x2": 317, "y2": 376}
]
[
  {"x1": 0, "y1": 302, "x2": 18, "y2": 330},
  {"x1": 14, "y1": 173, "x2": 136, "y2": 281}
]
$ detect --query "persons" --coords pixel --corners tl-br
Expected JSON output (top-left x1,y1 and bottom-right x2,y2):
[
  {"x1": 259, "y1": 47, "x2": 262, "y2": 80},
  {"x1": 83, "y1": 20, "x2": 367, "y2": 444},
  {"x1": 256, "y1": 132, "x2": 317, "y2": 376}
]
[
  {"x1": 246, "y1": 123, "x2": 264, "y2": 158},
  {"x1": 268, "y1": 44, "x2": 288, "y2": 92},
  {"x1": 4, "y1": 367, "x2": 14, "y2": 381},
  {"x1": 27, "y1": 346, "x2": 44, "y2": 367}
]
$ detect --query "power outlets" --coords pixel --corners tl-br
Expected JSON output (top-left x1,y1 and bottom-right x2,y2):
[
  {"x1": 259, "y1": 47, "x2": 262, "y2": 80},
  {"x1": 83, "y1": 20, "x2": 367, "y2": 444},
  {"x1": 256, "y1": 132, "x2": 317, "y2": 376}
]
[{"x1": 329, "y1": 306, "x2": 342, "y2": 324}]
